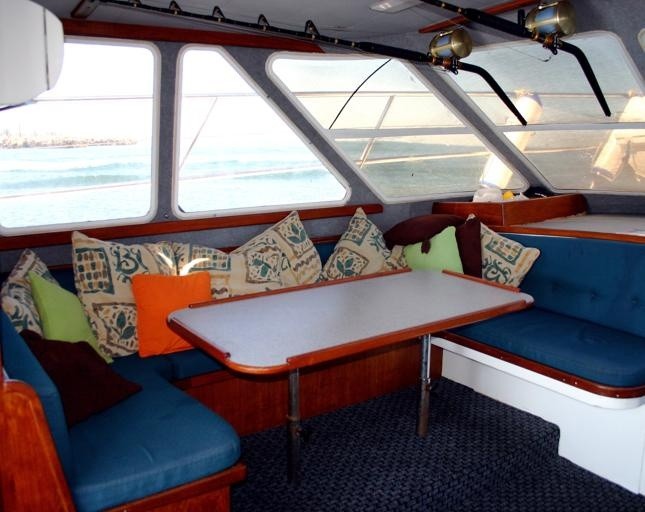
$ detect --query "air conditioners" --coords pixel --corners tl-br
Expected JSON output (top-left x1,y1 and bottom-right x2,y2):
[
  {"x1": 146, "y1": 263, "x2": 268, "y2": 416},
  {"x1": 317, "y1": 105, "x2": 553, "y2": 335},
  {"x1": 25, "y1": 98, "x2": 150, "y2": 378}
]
[{"x1": 0, "y1": 0, "x2": 64, "y2": 108}]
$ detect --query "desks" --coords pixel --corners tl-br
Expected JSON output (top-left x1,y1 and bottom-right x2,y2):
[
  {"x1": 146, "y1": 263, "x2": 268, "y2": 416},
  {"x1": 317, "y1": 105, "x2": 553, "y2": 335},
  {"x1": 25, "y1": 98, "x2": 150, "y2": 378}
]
[{"x1": 166, "y1": 268, "x2": 535, "y2": 440}]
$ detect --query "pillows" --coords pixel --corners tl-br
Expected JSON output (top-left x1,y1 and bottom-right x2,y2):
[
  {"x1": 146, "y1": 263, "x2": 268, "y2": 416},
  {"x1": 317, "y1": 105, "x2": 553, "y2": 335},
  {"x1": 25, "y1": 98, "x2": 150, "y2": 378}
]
[
  {"x1": 456, "y1": 217, "x2": 484, "y2": 279},
  {"x1": 388, "y1": 242, "x2": 407, "y2": 272},
  {"x1": 0, "y1": 248, "x2": 62, "y2": 332},
  {"x1": 20, "y1": 326, "x2": 140, "y2": 422},
  {"x1": 320, "y1": 207, "x2": 392, "y2": 281},
  {"x1": 72, "y1": 231, "x2": 178, "y2": 355},
  {"x1": 466, "y1": 213, "x2": 539, "y2": 292},
  {"x1": 31, "y1": 277, "x2": 111, "y2": 363},
  {"x1": 399, "y1": 225, "x2": 464, "y2": 274},
  {"x1": 229, "y1": 209, "x2": 320, "y2": 286},
  {"x1": 386, "y1": 213, "x2": 456, "y2": 253},
  {"x1": 170, "y1": 241, "x2": 285, "y2": 300},
  {"x1": 128, "y1": 270, "x2": 213, "y2": 357}
]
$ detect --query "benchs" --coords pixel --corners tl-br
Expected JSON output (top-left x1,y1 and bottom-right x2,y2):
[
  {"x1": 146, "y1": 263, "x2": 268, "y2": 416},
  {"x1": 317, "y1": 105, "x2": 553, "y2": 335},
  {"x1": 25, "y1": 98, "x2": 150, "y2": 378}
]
[
  {"x1": 76, "y1": 235, "x2": 442, "y2": 437},
  {"x1": 0, "y1": 287, "x2": 247, "y2": 512},
  {"x1": 434, "y1": 225, "x2": 645, "y2": 498}
]
